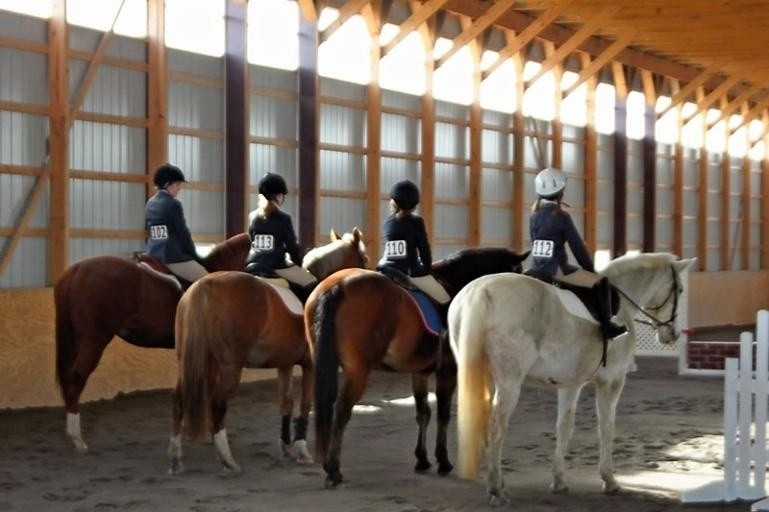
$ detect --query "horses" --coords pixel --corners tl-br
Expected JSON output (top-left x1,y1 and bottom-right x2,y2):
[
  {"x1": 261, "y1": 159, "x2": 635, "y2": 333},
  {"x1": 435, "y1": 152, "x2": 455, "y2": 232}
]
[
  {"x1": 166, "y1": 226, "x2": 372, "y2": 477},
  {"x1": 53, "y1": 232, "x2": 252, "y2": 451},
  {"x1": 446, "y1": 251, "x2": 699, "y2": 507},
  {"x1": 302, "y1": 246, "x2": 531, "y2": 489}
]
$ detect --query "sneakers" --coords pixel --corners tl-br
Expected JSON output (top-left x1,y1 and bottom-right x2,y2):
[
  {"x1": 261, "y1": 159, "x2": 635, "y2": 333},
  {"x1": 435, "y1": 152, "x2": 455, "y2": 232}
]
[{"x1": 605, "y1": 324, "x2": 627, "y2": 340}]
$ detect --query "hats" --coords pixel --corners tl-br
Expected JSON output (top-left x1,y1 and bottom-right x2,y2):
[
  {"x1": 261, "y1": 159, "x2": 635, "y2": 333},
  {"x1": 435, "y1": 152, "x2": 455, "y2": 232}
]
[
  {"x1": 532, "y1": 165, "x2": 569, "y2": 197},
  {"x1": 389, "y1": 179, "x2": 421, "y2": 210},
  {"x1": 257, "y1": 171, "x2": 289, "y2": 195},
  {"x1": 153, "y1": 162, "x2": 190, "y2": 186}
]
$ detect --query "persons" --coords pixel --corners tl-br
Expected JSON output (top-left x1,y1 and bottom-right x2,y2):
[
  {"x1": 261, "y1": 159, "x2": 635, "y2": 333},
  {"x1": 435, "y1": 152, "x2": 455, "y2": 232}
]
[
  {"x1": 377, "y1": 180, "x2": 454, "y2": 312},
  {"x1": 525, "y1": 166, "x2": 628, "y2": 340},
  {"x1": 244, "y1": 171, "x2": 319, "y2": 300},
  {"x1": 147, "y1": 162, "x2": 211, "y2": 284}
]
